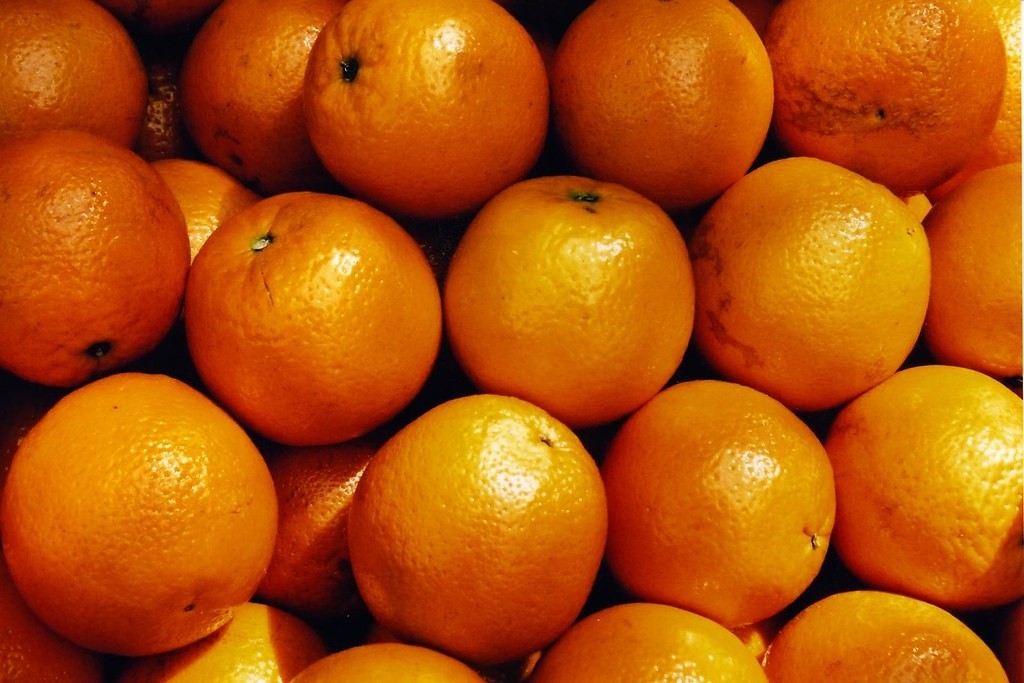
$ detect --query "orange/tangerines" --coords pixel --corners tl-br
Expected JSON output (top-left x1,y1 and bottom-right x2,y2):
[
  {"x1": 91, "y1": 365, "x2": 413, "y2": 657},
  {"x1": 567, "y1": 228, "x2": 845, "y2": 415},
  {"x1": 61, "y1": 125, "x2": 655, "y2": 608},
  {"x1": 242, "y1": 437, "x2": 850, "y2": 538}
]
[{"x1": 0, "y1": 0, "x2": 1023, "y2": 683}]
